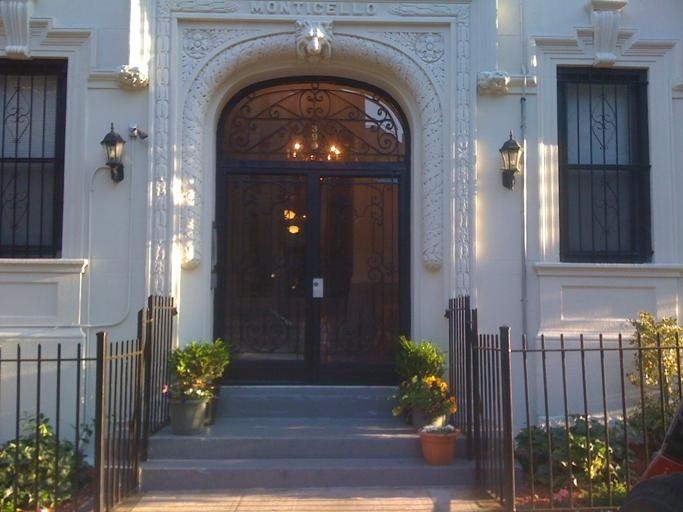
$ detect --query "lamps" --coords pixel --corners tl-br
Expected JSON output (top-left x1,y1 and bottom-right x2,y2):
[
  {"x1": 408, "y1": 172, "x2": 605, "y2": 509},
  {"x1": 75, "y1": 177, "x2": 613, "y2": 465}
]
[
  {"x1": 101, "y1": 121, "x2": 127, "y2": 183},
  {"x1": 497, "y1": 129, "x2": 524, "y2": 191}
]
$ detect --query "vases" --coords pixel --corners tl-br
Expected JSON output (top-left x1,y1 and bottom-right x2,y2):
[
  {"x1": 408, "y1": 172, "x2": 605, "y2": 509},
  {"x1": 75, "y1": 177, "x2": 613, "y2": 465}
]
[
  {"x1": 167, "y1": 397, "x2": 208, "y2": 435},
  {"x1": 420, "y1": 433, "x2": 458, "y2": 466}
]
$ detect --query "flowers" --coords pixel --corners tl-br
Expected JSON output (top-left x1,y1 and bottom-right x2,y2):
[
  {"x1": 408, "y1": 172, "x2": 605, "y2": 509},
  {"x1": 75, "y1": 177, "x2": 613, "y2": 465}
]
[
  {"x1": 388, "y1": 373, "x2": 459, "y2": 434},
  {"x1": 160, "y1": 377, "x2": 216, "y2": 405}
]
